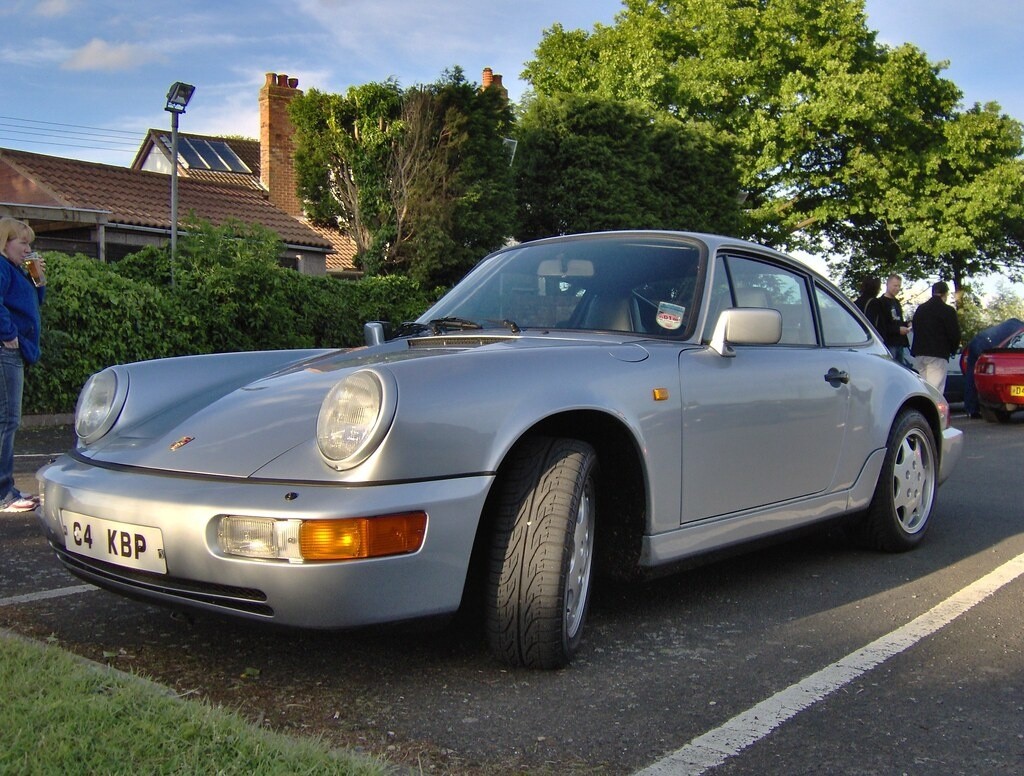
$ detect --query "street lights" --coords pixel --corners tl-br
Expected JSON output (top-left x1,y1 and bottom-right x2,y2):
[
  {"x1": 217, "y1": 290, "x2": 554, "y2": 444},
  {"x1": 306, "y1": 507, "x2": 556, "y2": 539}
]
[{"x1": 164, "y1": 81, "x2": 197, "y2": 289}]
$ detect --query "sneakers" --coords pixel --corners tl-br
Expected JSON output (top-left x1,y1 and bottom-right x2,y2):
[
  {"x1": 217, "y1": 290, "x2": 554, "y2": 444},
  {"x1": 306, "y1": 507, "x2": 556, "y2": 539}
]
[
  {"x1": 20, "y1": 491, "x2": 33, "y2": 500},
  {"x1": 0, "y1": 499, "x2": 36, "y2": 512}
]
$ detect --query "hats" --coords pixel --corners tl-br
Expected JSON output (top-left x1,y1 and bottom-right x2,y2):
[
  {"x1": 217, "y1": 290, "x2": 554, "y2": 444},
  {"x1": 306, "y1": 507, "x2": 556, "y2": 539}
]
[{"x1": 932, "y1": 280, "x2": 949, "y2": 293}]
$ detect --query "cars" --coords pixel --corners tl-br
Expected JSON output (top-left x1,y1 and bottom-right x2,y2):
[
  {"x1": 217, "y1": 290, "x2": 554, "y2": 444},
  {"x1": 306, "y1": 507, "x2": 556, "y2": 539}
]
[{"x1": 904, "y1": 317, "x2": 1024, "y2": 426}]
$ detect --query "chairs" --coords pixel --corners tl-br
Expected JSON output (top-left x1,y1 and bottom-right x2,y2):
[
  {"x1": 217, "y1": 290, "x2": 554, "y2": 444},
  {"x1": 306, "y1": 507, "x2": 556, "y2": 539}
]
[
  {"x1": 709, "y1": 288, "x2": 771, "y2": 340},
  {"x1": 583, "y1": 289, "x2": 647, "y2": 333}
]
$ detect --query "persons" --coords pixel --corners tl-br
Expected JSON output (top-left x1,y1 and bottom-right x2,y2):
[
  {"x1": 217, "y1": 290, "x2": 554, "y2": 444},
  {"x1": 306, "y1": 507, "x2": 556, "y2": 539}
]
[
  {"x1": 868, "y1": 274, "x2": 910, "y2": 361},
  {"x1": 910, "y1": 282, "x2": 960, "y2": 395},
  {"x1": 964, "y1": 318, "x2": 1024, "y2": 419},
  {"x1": 0, "y1": 217, "x2": 47, "y2": 512}
]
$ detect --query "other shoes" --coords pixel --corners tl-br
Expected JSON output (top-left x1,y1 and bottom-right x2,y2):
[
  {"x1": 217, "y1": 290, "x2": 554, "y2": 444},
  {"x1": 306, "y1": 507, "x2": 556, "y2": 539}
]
[{"x1": 967, "y1": 412, "x2": 982, "y2": 419}]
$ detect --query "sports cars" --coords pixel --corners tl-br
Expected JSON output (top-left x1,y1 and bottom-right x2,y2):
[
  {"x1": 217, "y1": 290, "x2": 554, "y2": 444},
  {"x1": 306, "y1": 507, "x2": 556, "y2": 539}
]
[{"x1": 32, "y1": 218, "x2": 968, "y2": 672}]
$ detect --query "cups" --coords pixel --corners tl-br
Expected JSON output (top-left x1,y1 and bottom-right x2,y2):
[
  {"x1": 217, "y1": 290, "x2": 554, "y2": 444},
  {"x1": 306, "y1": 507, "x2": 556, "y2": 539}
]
[{"x1": 25, "y1": 252, "x2": 47, "y2": 288}]
